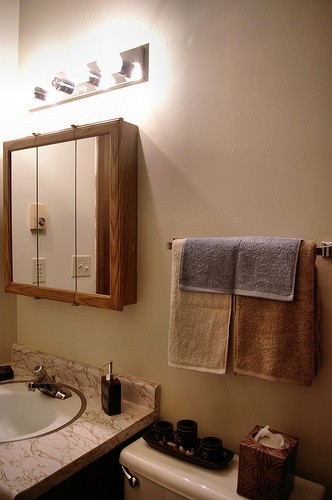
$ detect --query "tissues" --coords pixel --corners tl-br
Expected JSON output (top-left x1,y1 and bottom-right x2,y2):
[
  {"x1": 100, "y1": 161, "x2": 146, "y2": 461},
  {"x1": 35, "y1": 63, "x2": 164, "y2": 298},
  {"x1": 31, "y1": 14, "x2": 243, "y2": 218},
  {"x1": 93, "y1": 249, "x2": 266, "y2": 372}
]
[{"x1": 236, "y1": 424, "x2": 300, "y2": 500}]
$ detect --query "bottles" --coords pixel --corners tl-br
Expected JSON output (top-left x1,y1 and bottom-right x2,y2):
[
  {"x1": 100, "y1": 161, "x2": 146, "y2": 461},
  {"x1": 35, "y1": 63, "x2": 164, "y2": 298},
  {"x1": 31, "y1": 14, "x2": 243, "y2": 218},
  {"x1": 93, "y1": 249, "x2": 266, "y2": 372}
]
[{"x1": 101, "y1": 360, "x2": 122, "y2": 416}]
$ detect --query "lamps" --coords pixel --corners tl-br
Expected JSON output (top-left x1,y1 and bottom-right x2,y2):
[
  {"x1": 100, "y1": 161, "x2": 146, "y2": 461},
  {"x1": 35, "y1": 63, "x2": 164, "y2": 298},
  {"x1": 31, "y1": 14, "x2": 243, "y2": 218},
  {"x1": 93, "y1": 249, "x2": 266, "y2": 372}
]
[{"x1": 33, "y1": 53, "x2": 130, "y2": 101}]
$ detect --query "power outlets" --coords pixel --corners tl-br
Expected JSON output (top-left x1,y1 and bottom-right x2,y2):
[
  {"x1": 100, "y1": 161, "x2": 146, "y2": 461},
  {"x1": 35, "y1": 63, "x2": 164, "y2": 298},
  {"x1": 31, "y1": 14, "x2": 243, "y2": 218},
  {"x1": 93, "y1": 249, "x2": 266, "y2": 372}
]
[{"x1": 32, "y1": 258, "x2": 46, "y2": 283}]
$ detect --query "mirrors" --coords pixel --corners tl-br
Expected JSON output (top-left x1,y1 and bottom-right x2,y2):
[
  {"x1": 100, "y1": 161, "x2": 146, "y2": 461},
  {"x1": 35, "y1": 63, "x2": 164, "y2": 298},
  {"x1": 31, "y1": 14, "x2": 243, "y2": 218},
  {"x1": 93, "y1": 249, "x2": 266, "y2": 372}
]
[{"x1": 0, "y1": 117, "x2": 137, "y2": 312}]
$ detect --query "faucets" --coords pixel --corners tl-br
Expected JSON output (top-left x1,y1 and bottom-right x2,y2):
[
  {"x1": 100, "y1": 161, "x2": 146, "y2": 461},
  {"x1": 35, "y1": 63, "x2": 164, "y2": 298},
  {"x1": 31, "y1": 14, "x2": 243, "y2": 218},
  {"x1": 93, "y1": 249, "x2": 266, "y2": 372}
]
[{"x1": 28, "y1": 382, "x2": 62, "y2": 392}]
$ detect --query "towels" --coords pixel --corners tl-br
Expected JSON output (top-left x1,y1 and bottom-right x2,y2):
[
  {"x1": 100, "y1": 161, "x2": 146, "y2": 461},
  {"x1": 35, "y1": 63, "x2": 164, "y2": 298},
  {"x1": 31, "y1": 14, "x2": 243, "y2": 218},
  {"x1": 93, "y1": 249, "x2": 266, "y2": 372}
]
[
  {"x1": 179, "y1": 236, "x2": 304, "y2": 302},
  {"x1": 167, "y1": 238, "x2": 235, "y2": 375},
  {"x1": 234, "y1": 241, "x2": 320, "y2": 387}
]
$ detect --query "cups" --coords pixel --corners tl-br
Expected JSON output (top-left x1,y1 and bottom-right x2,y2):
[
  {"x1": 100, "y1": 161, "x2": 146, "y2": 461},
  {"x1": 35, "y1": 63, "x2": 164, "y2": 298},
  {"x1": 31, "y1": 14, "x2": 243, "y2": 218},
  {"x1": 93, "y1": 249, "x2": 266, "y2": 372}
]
[
  {"x1": 198, "y1": 436, "x2": 223, "y2": 461},
  {"x1": 153, "y1": 421, "x2": 173, "y2": 444},
  {"x1": 176, "y1": 419, "x2": 198, "y2": 449}
]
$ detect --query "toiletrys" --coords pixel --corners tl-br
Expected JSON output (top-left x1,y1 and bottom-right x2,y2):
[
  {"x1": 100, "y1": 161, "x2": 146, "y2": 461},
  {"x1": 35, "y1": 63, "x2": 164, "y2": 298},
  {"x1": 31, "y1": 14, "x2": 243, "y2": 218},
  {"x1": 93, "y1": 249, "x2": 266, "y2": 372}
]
[{"x1": 100, "y1": 360, "x2": 121, "y2": 416}]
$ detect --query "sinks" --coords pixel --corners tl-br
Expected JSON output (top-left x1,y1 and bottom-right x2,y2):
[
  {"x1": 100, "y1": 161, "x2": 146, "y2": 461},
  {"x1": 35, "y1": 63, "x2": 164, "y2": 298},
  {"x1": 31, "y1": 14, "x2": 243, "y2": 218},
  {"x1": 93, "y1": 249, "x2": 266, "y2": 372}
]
[{"x1": 0, "y1": 380, "x2": 81, "y2": 442}]
